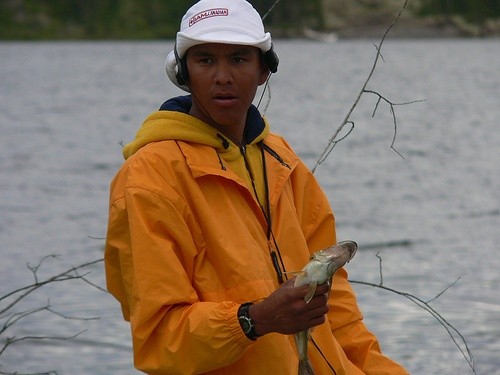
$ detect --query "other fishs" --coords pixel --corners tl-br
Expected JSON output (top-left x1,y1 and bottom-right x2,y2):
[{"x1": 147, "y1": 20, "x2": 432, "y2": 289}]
[{"x1": 283, "y1": 240, "x2": 358, "y2": 375}]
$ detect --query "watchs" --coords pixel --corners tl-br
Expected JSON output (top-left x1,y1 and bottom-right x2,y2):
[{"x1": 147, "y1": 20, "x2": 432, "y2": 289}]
[{"x1": 237, "y1": 302, "x2": 262, "y2": 341}]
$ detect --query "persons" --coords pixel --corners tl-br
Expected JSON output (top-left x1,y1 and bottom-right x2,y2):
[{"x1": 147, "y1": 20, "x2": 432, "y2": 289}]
[{"x1": 104, "y1": 0, "x2": 412, "y2": 375}]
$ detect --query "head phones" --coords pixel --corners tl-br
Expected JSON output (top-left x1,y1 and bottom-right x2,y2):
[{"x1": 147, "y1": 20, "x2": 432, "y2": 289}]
[{"x1": 175, "y1": 39, "x2": 279, "y2": 86}]
